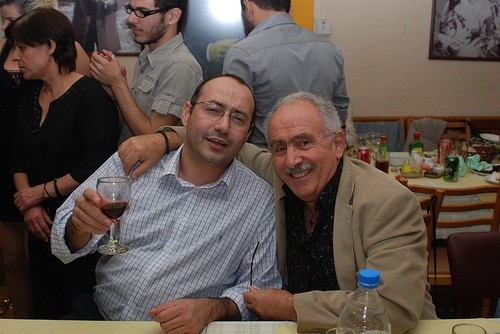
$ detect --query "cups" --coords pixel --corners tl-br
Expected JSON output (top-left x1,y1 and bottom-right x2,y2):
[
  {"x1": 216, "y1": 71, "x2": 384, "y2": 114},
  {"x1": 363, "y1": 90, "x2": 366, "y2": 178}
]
[
  {"x1": 450, "y1": 321, "x2": 486, "y2": 333},
  {"x1": 373, "y1": 150, "x2": 389, "y2": 175}
]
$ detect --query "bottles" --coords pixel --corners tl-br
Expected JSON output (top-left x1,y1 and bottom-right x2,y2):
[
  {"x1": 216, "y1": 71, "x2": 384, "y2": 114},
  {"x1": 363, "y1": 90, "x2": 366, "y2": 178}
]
[
  {"x1": 380, "y1": 136, "x2": 388, "y2": 158},
  {"x1": 336, "y1": 271, "x2": 391, "y2": 333},
  {"x1": 409, "y1": 131, "x2": 422, "y2": 166}
]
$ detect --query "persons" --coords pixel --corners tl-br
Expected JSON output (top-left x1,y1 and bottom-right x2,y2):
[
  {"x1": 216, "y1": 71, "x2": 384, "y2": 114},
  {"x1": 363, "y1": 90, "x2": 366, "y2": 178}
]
[
  {"x1": 222, "y1": 1, "x2": 350, "y2": 147},
  {"x1": 115, "y1": 89, "x2": 438, "y2": 334},
  {"x1": 87, "y1": 0, "x2": 204, "y2": 149},
  {"x1": 52, "y1": 72, "x2": 284, "y2": 333},
  {"x1": 0, "y1": 0, "x2": 90, "y2": 321},
  {"x1": 0, "y1": 5, "x2": 124, "y2": 323}
]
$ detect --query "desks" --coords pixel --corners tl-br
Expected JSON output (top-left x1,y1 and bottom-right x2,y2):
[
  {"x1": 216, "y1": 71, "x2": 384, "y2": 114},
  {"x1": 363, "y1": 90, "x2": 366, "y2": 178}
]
[{"x1": 0, "y1": 319, "x2": 499, "y2": 334}]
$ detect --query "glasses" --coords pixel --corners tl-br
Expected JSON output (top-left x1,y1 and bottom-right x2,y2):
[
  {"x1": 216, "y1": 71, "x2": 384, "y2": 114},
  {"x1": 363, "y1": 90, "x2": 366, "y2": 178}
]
[
  {"x1": 191, "y1": 100, "x2": 255, "y2": 127},
  {"x1": 123, "y1": 3, "x2": 170, "y2": 17}
]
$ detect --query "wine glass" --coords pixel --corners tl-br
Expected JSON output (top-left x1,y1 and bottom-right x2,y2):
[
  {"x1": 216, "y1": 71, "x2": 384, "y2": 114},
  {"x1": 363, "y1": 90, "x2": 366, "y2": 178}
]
[
  {"x1": 95, "y1": 175, "x2": 130, "y2": 255},
  {"x1": 350, "y1": 129, "x2": 378, "y2": 157}
]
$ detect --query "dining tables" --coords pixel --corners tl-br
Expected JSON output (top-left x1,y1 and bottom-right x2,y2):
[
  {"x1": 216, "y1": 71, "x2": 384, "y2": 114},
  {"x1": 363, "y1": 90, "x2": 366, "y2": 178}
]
[{"x1": 348, "y1": 152, "x2": 500, "y2": 242}]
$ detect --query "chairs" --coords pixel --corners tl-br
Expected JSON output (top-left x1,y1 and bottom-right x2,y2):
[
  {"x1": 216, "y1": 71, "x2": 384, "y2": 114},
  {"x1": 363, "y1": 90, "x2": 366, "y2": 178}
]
[
  {"x1": 352, "y1": 117, "x2": 406, "y2": 151},
  {"x1": 470, "y1": 115, "x2": 500, "y2": 141},
  {"x1": 407, "y1": 115, "x2": 471, "y2": 149},
  {"x1": 400, "y1": 177, "x2": 500, "y2": 289},
  {"x1": 447, "y1": 232, "x2": 500, "y2": 319},
  {"x1": 418, "y1": 187, "x2": 446, "y2": 287}
]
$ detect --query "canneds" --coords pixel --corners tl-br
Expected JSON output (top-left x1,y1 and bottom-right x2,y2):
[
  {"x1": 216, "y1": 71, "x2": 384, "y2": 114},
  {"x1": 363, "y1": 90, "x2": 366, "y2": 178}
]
[
  {"x1": 443, "y1": 156, "x2": 460, "y2": 183},
  {"x1": 357, "y1": 146, "x2": 371, "y2": 165}
]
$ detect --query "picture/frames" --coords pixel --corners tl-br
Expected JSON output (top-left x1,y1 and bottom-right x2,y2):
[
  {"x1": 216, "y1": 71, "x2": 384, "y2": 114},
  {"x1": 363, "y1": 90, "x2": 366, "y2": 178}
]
[
  {"x1": 92, "y1": 0, "x2": 145, "y2": 56},
  {"x1": 429, "y1": 1, "x2": 500, "y2": 62}
]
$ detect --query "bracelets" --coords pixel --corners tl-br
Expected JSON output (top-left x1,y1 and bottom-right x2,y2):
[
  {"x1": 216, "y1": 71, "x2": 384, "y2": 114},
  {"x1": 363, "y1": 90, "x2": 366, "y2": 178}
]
[
  {"x1": 43, "y1": 178, "x2": 64, "y2": 200},
  {"x1": 156, "y1": 130, "x2": 169, "y2": 155}
]
